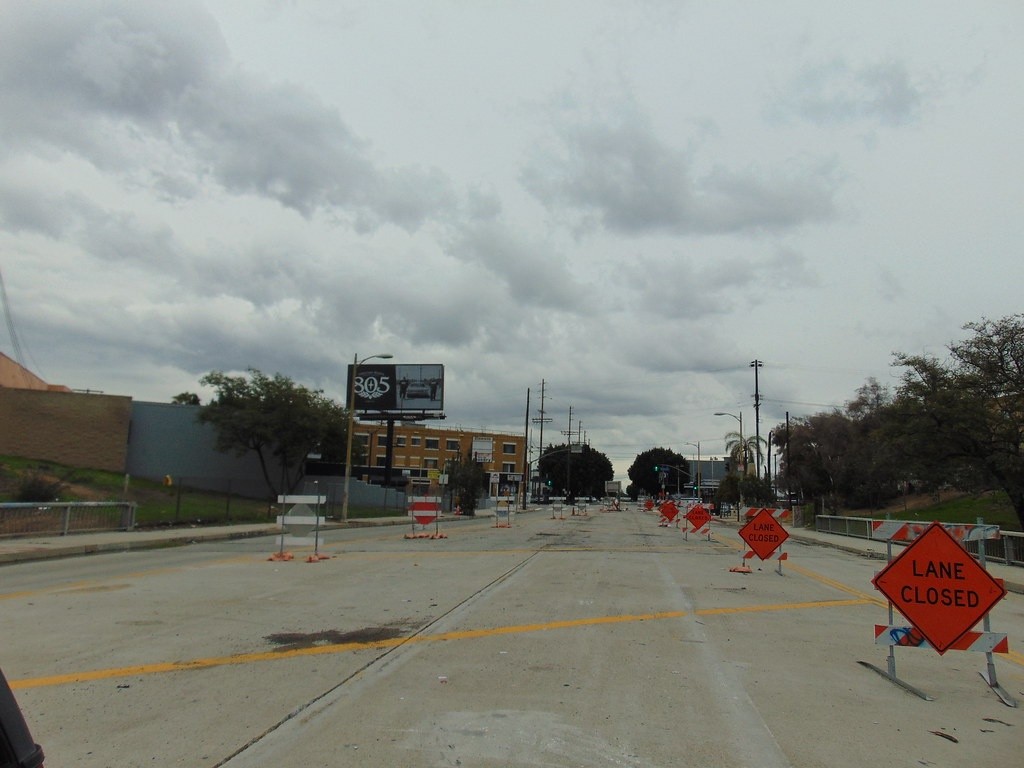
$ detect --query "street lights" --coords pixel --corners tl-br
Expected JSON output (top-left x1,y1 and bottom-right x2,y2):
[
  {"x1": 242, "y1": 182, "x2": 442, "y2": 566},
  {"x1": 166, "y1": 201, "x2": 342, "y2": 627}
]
[
  {"x1": 341, "y1": 350, "x2": 394, "y2": 520},
  {"x1": 714, "y1": 409, "x2": 744, "y2": 505},
  {"x1": 687, "y1": 439, "x2": 702, "y2": 504}
]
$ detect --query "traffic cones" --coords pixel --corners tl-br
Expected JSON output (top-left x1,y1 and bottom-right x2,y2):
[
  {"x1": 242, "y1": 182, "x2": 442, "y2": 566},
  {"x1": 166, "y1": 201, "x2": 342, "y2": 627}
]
[{"x1": 456, "y1": 504, "x2": 460, "y2": 515}]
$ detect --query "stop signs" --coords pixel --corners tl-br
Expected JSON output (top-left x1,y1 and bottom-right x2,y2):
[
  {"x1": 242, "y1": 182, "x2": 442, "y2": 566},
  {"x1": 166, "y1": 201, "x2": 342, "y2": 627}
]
[{"x1": 407, "y1": 496, "x2": 444, "y2": 526}]
